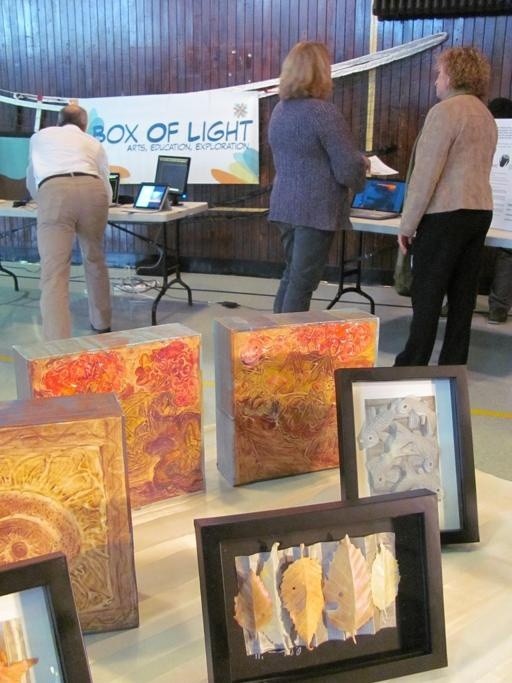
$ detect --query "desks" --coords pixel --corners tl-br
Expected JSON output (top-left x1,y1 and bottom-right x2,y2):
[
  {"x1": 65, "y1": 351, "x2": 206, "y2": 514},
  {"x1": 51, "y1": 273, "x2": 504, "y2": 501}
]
[
  {"x1": 0, "y1": 199, "x2": 209, "y2": 325},
  {"x1": 325, "y1": 211, "x2": 512, "y2": 314},
  {"x1": 0, "y1": 443, "x2": 512, "y2": 683}
]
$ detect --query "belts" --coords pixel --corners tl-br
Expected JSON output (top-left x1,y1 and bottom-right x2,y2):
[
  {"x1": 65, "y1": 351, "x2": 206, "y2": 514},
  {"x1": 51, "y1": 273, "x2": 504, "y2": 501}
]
[{"x1": 38, "y1": 173, "x2": 98, "y2": 188}]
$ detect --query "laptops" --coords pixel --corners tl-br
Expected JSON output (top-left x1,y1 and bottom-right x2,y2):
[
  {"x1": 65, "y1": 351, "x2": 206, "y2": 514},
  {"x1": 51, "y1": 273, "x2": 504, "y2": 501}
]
[
  {"x1": 109, "y1": 173, "x2": 120, "y2": 207},
  {"x1": 346, "y1": 178, "x2": 408, "y2": 220}
]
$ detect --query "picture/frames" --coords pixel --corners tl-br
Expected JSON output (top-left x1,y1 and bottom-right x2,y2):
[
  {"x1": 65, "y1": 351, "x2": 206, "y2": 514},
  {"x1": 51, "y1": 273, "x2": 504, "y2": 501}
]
[
  {"x1": 334, "y1": 363, "x2": 481, "y2": 542},
  {"x1": 193, "y1": 487, "x2": 449, "y2": 682},
  {"x1": 0, "y1": 550, "x2": 93, "y2": 681}
]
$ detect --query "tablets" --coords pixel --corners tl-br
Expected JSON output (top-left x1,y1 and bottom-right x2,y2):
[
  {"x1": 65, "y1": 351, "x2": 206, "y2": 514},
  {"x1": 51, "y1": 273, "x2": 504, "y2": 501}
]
[{"x1": 131, "y1": 184, "x2": 169, "y2": 209}]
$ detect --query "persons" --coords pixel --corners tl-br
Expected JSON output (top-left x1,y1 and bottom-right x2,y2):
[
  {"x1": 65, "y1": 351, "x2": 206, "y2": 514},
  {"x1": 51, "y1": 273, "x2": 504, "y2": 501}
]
[
  {"x1": 25, "y1": 104, "x2": 113, "y2": 342},
  {"x1": 394, "y1": 46, "x2": 499, "y2": 367},
  {"x1": 266, "y1": 40, "x2": 371, "y2": 313}
]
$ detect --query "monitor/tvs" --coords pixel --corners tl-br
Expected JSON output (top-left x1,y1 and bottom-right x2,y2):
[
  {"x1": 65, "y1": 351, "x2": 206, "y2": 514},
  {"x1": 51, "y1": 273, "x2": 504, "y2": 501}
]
[{"x1": 153, "y1": 155, "x2": 191, "y2": 206}]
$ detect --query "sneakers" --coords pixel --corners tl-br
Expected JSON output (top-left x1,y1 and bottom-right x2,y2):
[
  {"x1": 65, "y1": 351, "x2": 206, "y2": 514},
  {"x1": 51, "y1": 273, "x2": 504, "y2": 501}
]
[{"x1": 488, "y1": 309, "x2": 507, "y2": 323}]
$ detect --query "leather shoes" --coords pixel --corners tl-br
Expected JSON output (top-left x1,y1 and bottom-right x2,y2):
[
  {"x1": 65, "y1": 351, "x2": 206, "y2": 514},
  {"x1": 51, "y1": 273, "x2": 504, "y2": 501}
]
[{"x1": 91, "y1": 324, "x2": 111, "y2": 334}]
